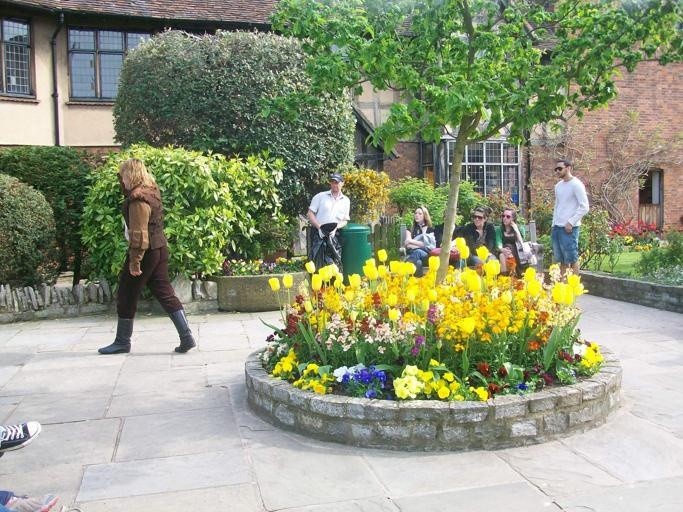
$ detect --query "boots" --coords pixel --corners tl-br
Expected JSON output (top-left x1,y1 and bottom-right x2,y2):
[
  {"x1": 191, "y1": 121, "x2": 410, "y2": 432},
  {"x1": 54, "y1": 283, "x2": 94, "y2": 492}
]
[
  {"x1": 168, "y1": 309, "x2": 197, "y2": 353},
  {"x1": 98, "y1": 318, "x2": 133, "y2": 355}
]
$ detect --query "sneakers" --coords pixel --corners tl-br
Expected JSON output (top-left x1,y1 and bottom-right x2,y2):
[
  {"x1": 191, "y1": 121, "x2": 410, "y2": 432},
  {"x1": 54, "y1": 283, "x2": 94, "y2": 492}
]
[
  {"x1": 0, "y1": 420, "x2": 42, "y2": 453},
  {"x1": 4, "y1": 494, "x2": 61, "y2": 512}
]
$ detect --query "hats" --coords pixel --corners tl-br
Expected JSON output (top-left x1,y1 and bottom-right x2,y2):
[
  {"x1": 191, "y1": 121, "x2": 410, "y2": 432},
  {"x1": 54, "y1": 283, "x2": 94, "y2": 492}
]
[{"x1": 328, "y1": 173, "x2": 344, "y2": 184}]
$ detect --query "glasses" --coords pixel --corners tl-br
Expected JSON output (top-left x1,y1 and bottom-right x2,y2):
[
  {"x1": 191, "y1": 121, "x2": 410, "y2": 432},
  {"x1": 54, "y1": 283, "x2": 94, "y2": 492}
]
[
  {"x1": 502, "y1": 214, "x2": 510, "y2": 218},
  {"x1": 554, "y1": 167, "x2": 562, "y2": 171},
  {"x1": 474, "y1": 215, "x2": 484, "y2": 220}
]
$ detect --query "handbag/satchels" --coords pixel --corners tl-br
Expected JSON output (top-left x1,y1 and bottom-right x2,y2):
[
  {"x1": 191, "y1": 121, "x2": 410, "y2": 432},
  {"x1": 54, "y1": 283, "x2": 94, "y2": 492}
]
[
  {"x1": 407, "y1": 232, "x2": 435, "y2": 252},
  {"x1": 514, "y1": 241, "x2": 532, "y2": 264}
]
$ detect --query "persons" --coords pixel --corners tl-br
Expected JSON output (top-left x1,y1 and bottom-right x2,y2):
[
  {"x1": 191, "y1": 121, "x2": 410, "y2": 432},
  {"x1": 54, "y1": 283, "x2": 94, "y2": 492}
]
[
  {"x1": 308, "y1": 175, "x2": 351, "y2": 242},
  {"x1": 404, "y1": 204, "x2": 438, "y2": 277},
  {"x1": 434, "y1": 209, "x2": 468, "y2": 270},
  {"x1": 97, "y1": 158, "x2": 196, "y2": 354},
  {"x1": 464, "y1": 206, "x2": 503, "y2": 267},
  {"x1": 1, "y1": 421, "x2": 42, "y2": 457},
  {"x1": 0, "y1": 490, "x2": 61, "y2": 512},
  {"x1": 495, "y1": 208, "x2": 526, "y2": 279},
  {"x1": 552, "y1": 159, "x2": 589, "y2": 276}
]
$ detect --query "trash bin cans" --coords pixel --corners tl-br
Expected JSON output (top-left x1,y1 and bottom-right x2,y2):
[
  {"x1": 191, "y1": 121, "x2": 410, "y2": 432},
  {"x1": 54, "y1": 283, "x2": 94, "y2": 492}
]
[{"x1": 341, "y1": 223, "x2": 372, "y2": 286}]
